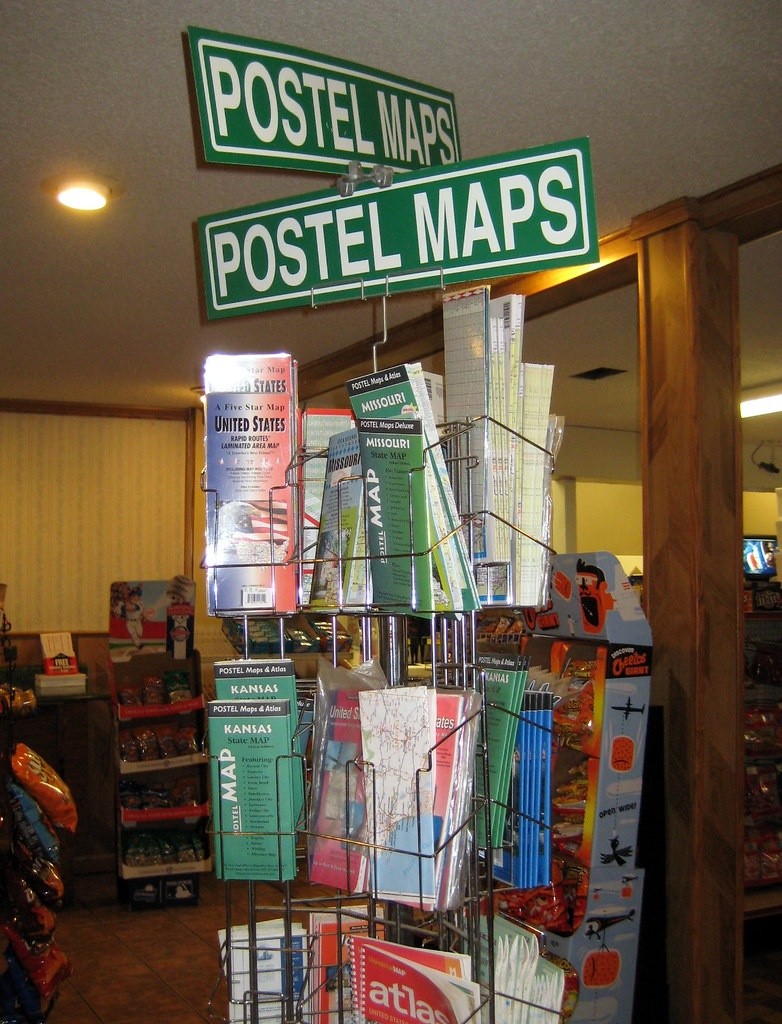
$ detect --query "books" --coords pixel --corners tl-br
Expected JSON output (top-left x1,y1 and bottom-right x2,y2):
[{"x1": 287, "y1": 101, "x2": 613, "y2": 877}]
[
  {"x1": 204, "y1": 284, "x2": 566, "y2": 618},
  {"x1": 217, "y1": 903, "x2": 565, "y2": 1024},
  {"x1": 206, "y1": 654, "x2": 555, "y2": 913}
]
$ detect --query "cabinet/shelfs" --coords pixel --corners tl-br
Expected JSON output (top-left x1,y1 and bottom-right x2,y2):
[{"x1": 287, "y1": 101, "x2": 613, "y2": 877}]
[
  {"x1": 200, "y1": 417, "x2": 571, "y2": 1024},
  {"x1": 0, "y1": 684, "x2": 116, "y2": 907},
  {"x1": 108, "y1": 647, "x2": 211, "y2": 911},
  {"x1": 742, "y1": 581, "x2": 782, "y2": 920}
]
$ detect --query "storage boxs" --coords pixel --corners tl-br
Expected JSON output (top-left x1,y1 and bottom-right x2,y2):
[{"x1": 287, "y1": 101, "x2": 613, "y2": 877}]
[
  {"x1": 221, "y1": 615, "x2": 354, "y2": 655},
  {"x1": 493, "y1": 627, "x2": 656, "y2": 1023}
]
